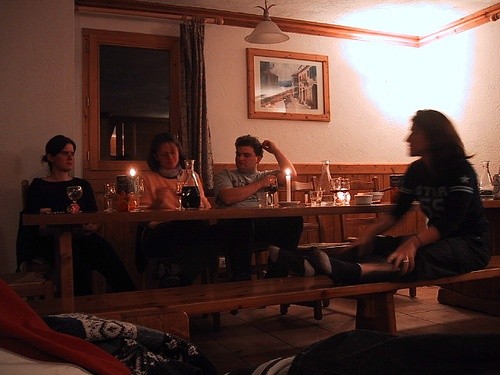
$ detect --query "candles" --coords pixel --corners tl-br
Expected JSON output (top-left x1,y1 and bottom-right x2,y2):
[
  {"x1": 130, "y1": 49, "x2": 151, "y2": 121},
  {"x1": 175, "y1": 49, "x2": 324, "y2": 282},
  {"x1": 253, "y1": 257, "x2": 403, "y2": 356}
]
[{"x1": 285, "y1": 169, "x2": 292, "y2": 201}]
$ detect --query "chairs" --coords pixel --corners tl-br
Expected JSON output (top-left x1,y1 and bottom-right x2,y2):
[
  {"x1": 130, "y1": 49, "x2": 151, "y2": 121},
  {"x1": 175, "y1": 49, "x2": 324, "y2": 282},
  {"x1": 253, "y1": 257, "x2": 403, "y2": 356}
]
[{"x1": 273, "y1": 176, "x2": 383, "y2": 245}]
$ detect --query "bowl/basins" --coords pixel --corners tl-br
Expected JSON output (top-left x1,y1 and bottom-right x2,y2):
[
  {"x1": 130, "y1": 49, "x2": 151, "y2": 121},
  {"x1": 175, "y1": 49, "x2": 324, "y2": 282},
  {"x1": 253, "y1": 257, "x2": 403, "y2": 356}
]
[
  {"x1": 368, "y1": 192, "x2": 385, "y2": 202},
  {"x1": 354, "y1": 195, "x2": 373, "y2": 204}
]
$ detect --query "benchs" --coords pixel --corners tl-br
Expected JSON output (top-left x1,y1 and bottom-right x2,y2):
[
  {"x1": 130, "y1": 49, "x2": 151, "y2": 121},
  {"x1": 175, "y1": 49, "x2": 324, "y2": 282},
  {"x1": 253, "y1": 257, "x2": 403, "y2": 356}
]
[{"x1": 5, "y1": 240, "x2": 499, "y2": 342}]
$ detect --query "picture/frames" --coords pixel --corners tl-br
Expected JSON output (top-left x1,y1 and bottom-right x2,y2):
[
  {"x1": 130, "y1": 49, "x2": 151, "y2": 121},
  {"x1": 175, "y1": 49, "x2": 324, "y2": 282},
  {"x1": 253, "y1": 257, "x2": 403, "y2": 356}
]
[{"x1": 247, "y1": 48, "x2": 331, "y2": 122}]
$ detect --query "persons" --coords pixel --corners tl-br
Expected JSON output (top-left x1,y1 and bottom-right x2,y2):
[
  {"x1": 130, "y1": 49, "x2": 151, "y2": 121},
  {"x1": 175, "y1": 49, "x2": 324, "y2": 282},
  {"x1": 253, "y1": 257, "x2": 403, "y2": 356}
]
[
  {"x1": 270, "y1": 110, "x2": 491, "y2": 286},
  {"x1": 24, "y1": 135, "x2": 136, "y2": 296},
  {"x1": 128, "y1": 133, "x2": 218, "y2": 285},
  {"x1": 213, "y1": 135, "x2": 304, "y2": 281}
]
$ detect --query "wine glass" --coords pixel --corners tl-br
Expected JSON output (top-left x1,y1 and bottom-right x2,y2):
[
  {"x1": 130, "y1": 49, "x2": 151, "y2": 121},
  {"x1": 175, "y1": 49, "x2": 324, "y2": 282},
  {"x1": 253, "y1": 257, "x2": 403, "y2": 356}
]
[
  {"x1": 67, "y1": 186, "x2": 82, "y2": 203},
  {"x1": 132, "y1": 179, "x2": 144, "y2": 211},
  {"x1": 103, "y1": 182, "x2": 117, "y2": 213},
  {"x1": 329, "y1": 177, "x2": 350, "y2": 207},
  {"x1": 265, "y1": 176, "x2": 278, "y2": 207}
]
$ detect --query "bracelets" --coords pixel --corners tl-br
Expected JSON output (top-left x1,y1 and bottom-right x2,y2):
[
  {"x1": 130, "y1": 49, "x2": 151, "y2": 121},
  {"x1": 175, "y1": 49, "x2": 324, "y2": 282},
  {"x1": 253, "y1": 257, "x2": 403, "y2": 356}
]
[{"x1": 416, "y1": 234, "x2": 424, "y2": 245}]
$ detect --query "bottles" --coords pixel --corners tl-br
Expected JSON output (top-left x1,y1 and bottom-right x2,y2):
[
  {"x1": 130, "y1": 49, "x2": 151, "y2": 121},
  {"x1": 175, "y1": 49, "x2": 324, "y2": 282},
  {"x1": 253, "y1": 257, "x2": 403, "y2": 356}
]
[
  {"x1": 318, "y1": 160, "x2": 335, "y2": 206},
  {"x1": 480, "y1": 162, "x2": 492, "y2": 189},
  {"x1": 181, "y1": 159, "x2": 201, "y2": 210},
  {"x1": 128, "y1": 192, "x2": 138, "y2": 211}
]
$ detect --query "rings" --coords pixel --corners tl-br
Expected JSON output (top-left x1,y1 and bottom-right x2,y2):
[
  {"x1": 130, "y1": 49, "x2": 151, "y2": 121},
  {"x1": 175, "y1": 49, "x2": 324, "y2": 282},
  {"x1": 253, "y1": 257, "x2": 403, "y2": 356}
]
[{"x1": 403, "y1": 256, "x2": 409, "y2": 263}]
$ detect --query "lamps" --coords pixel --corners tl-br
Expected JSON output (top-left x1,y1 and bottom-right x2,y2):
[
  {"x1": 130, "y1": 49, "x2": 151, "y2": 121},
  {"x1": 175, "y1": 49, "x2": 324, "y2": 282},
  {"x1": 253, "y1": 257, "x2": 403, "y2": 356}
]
[{"x1": 244, "y1": 0, "x2": 290, "y2": 44}]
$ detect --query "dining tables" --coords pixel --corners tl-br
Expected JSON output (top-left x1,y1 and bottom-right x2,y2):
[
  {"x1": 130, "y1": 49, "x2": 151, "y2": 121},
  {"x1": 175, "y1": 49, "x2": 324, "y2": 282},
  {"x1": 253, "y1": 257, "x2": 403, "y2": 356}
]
[{"x1": 21, "y1": 198, "x2": 419, "y2": 292}]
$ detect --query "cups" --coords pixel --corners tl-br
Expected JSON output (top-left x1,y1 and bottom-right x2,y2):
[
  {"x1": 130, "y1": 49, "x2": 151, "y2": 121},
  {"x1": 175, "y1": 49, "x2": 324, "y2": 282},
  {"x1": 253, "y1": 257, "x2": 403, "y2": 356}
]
[
  {"x1": 310, "y1": 190, "x2": 322, "y2": 207},
  {"x1": 257, "y1": 192, "x2": 269, "y2": 208}
]
